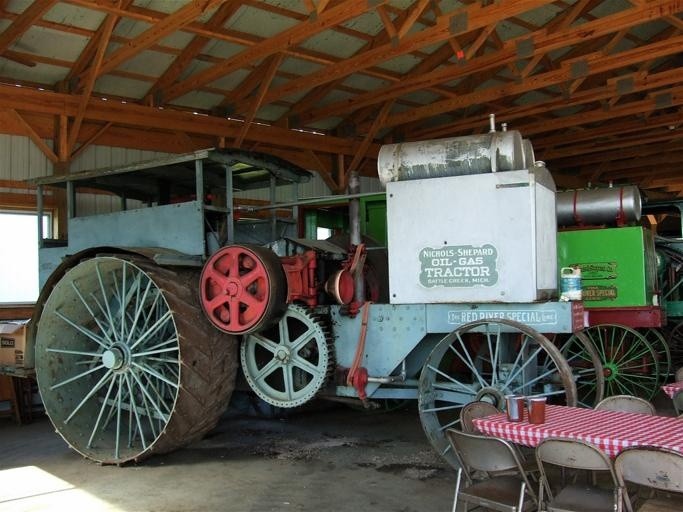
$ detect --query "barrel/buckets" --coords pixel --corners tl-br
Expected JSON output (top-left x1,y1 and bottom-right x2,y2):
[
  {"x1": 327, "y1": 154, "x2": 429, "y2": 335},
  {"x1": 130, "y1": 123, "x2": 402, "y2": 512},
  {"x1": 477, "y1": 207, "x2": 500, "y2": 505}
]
[{"x1": 560, "y1": 266, "x2": 583, "y2": 302}]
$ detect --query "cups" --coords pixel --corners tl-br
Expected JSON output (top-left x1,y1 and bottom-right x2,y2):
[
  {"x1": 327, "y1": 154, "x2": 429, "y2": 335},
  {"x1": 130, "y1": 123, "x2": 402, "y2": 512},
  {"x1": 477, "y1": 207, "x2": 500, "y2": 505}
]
[
  {"x1": 504, "y1": 394, "x2": 526, "y2": 422},
  {"x1": 526, "y1": 395, "x2": 547, "y2": 424}
]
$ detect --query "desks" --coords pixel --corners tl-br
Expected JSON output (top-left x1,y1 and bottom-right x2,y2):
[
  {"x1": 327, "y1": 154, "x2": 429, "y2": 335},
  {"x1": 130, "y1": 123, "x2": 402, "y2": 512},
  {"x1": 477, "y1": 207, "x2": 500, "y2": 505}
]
[{"x1": 1, "y1": 372, "x2": 39, "y2": 427}]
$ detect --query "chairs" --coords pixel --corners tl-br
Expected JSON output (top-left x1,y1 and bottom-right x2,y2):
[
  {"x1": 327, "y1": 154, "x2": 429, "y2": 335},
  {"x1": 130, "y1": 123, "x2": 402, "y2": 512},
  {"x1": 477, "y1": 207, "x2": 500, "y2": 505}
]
[{"x1": 443, "y1": 389, "x2": 683, "y2": 512}]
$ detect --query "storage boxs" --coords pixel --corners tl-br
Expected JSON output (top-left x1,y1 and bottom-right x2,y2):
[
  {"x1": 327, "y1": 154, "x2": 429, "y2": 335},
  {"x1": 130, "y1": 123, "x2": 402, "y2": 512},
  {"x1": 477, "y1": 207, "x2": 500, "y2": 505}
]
[{"x1": 0, "y1": 320, "x2": 36, "y2": 370}]
